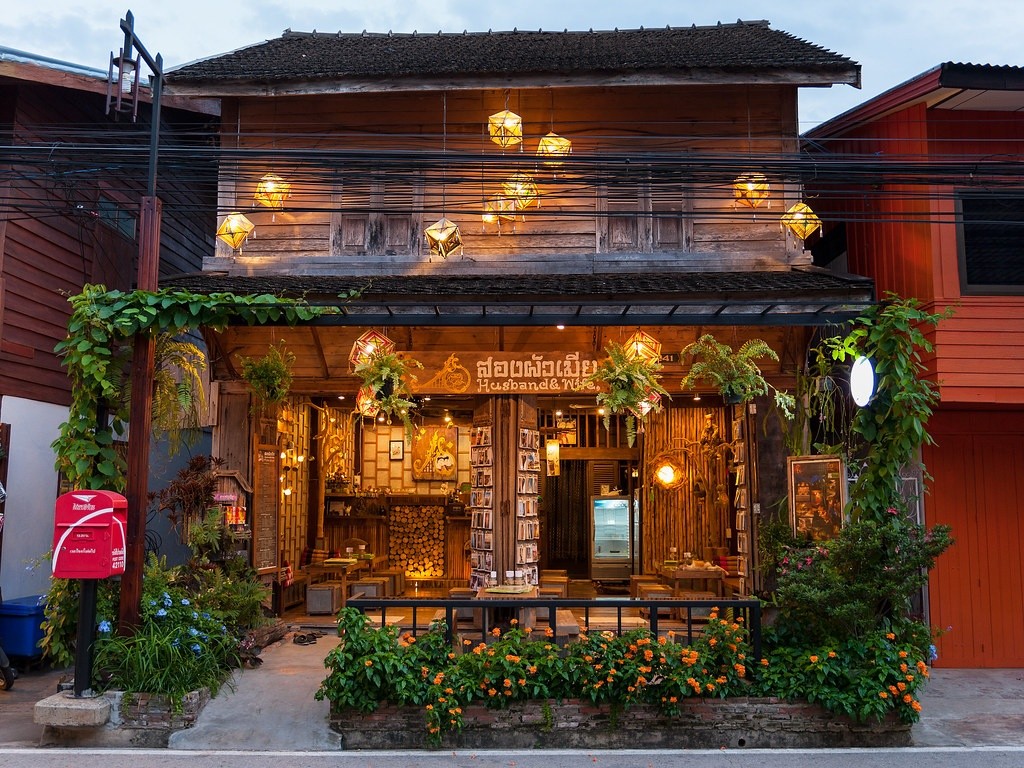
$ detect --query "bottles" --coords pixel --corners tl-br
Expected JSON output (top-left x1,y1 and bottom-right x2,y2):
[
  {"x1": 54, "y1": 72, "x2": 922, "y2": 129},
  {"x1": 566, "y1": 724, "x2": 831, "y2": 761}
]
[
  {"x1": 598, "y1": 546, "x2": 601, "y2": 553},
  {"x1": 353, "y1": 486, "x2": 390, "y2": 498},
  {"x1": 326, "y1": 473, "x2": 352, "y2": 494},
  {"x1": 380, "y1": 506, "x2": 386, "y2": 516}
]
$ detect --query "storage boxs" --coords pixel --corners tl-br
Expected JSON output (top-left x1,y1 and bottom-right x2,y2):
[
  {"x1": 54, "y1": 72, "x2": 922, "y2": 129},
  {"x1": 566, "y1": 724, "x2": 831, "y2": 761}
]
[{"x1": 0, "y1": 593, "x2": 49, "y2": 674}]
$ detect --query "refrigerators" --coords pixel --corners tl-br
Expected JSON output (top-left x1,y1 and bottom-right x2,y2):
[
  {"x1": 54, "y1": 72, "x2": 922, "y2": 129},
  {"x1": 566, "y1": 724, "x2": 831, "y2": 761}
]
[{"x1": 590, "y1": 495, "x2": 632, "y2": 580}]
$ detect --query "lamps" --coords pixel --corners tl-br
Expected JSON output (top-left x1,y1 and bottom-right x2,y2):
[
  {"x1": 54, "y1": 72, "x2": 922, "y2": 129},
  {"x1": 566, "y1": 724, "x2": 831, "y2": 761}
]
[
  {"x1": 445, "y1": 413, "x2": 451, "y2": 422},
  {"x1": 620, "y1": 325, "x2": 662, "y2": 419},
  {"x1": 693, "y1": 393, "x2": 700, "y2": 401},
  {"x1": 425, "y1": 87, "x2": 573, "y2": 263},
  {"x1": 347, "y1": 326, "x2": 430, "y2": 421},
  {"x1": 732, "y1": 87, "x2": 823, "y2": 255},
  {"x1": 216, "y1": 97, "x2": 292, "y2": 257}
]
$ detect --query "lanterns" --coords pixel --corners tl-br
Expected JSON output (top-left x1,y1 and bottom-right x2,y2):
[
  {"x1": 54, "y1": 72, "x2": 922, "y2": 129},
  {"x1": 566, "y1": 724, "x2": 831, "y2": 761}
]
[
  {"x1": 626, "y1": 386, "x2": 662, "y2": 433},
  {"x1": 423, "y1": 218, "x2": 464, "y2": 263},
  {"x1": 732, "y1": 171, "x2": 771, "y2": 221},
  {"x1": 622, "y1": 328, "x2": 662, "y2": 371},
  {"x1": 355, "y1": 382, "x2": 391, "y2": 432},
  {"x1": 215, "y1": 211, "x2": 256, "y2": 255},
  {"x1": 482, "y1": 192, "x2": 517, "y2": 237},
  {"x1": 780, "y1": 201, "x2": 823, "y2": 254},
  {"x1": 252, "y1": 173, "x2": 292, "y2": 223},
  {"x1": 488, "y1": 110, "x2": 524, "y2": 156},
  {"x1": 503, "y1": 171, "x2": 541, "y2": 222},
  {"x1": 348, "y1": 327, "x2": 396, "y2": 378},
  {"x1": 534, "y1": 131, "x2": 571, "y2": 180}
]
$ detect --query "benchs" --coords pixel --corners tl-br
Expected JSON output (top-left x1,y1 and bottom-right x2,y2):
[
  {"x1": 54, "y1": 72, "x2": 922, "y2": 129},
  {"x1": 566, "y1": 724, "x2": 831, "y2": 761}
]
[
  {"x1": 723, "y1": 577, "x2": 739, "y2": 598},
  {"x1": 284, "y1": 570, "x2": 306, "y2": 607}
]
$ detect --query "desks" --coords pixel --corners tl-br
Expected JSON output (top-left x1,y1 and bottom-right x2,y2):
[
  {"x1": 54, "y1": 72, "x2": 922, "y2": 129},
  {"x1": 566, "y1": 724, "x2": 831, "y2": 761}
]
[
  {"x1": 301, "y1": 554, "x2": 389, "y2": 608},
  {"x1": 477, "y1": 587, "x2": 537, "y2": 632},
  {"x1": 652, "y1": 558, "x2": 723, "y2": 623}
]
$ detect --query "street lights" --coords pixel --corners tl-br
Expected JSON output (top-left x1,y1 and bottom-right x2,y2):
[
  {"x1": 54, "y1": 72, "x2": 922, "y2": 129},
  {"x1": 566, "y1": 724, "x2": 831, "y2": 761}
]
[{"x1": 104, "y1": 11, "x2": 168, "y2": 643}]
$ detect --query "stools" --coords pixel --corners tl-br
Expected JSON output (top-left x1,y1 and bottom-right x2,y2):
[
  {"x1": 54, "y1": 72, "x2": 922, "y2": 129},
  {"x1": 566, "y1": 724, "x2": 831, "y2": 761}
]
[
  {"x1": 536, "y1": 570, "x2": 568, "y2": 620},
  {"x1": 629, "y1": 575, "x2": 717, "y2": 624},
  {"x1": 449, "y1": 587, "x2": 475, "y2": 619},
  {"x1": 306, "y1": 569, "x2": 406, "y2": 616}
]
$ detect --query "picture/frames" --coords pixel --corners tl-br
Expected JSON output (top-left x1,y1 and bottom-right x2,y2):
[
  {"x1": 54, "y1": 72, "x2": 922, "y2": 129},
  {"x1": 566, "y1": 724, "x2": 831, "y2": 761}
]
[{"x1": 389, "y1": 440, "x2": 404, "y2": 459}]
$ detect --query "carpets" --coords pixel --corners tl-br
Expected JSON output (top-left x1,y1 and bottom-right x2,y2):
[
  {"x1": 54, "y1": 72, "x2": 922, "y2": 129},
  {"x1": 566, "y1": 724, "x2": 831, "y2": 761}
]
[
  {"x1": 333, "y1": 616, "x2": 406, "y2": 623},
  {"x1": 580, "y1": 616, "x2": 649, "y2": 624}
]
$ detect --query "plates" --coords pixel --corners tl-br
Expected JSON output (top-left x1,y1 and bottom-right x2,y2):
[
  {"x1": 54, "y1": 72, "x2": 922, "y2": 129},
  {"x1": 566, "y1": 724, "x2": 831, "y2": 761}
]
[{"x1": 610, "y1": 551, "x2": 620, "y2": 553}]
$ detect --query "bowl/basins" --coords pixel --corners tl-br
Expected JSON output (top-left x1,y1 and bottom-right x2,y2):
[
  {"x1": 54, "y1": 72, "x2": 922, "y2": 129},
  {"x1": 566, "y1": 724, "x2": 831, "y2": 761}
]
[{"x1": 664, "y1": 561, "x2": 677, "y2": 568}]
